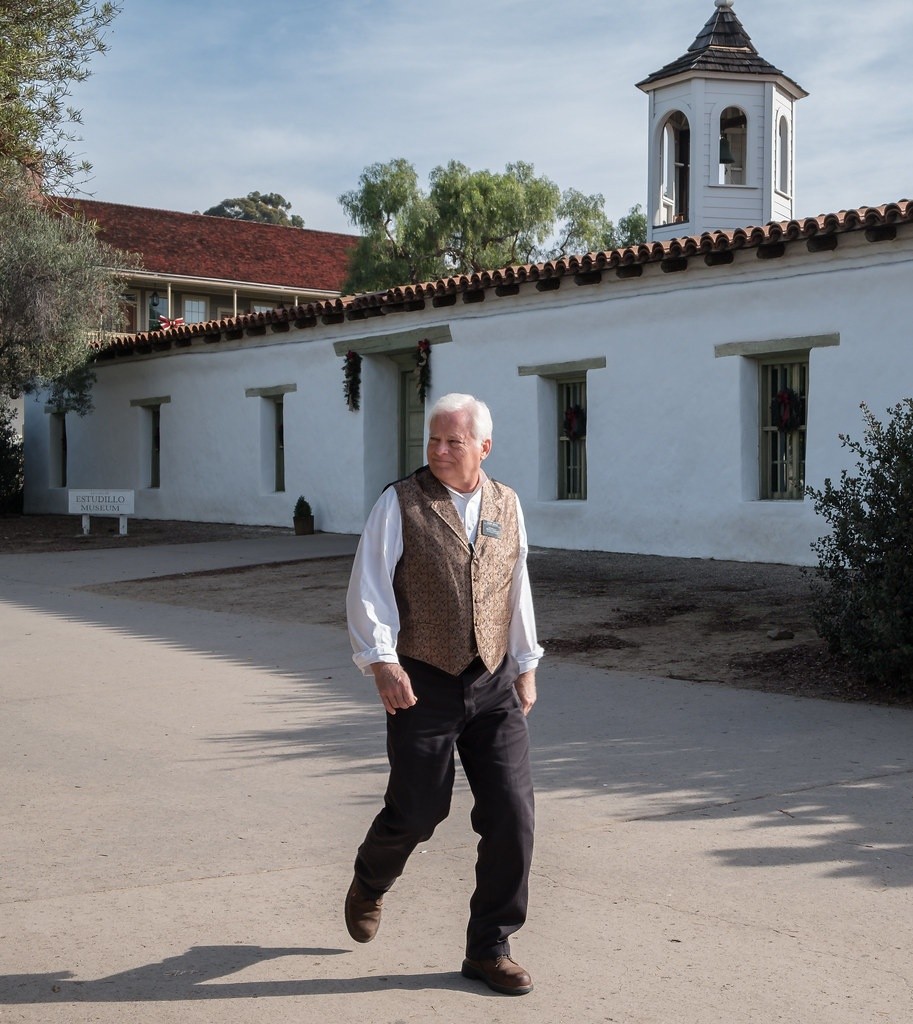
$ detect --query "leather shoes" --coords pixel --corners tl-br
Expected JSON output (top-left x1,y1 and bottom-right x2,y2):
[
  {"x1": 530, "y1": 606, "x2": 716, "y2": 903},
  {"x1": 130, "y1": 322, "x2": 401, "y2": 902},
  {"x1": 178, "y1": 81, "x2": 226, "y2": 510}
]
[
  {"x1": 345, "y1": 861, "x2": 397, "y2": 942},
  {"x1": 461, "y1": 951, "x2": 534, "y2": 995}
]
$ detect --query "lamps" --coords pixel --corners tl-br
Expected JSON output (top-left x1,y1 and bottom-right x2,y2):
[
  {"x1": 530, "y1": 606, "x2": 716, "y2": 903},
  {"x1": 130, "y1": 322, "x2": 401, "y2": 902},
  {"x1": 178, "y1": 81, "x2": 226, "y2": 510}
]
[
  {"x1": 279, "y1": 295, "x2": 285, "y2": 310},
  {"x1": 151, "y1": 283, "x2": 160, "y2": 307}
]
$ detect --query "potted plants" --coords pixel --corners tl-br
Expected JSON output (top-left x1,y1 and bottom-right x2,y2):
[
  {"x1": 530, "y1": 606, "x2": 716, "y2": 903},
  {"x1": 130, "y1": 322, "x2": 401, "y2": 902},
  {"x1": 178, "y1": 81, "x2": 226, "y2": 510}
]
[{"x1": 292, "y1": 497, "x2": 314, "y2": 537}]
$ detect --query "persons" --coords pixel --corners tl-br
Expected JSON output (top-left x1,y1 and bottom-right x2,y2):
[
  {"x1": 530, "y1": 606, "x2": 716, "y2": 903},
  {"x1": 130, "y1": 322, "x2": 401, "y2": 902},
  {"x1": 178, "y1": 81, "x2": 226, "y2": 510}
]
[{"x1": 346, "y1": 393, "x2": 545, "y2": 996}]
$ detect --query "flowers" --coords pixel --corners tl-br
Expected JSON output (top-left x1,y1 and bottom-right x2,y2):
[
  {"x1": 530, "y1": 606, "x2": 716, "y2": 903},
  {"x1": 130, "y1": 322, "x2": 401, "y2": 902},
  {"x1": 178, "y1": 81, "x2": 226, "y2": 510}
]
[
  {"x1": 412, "y1": 337, "x2": 432, "y2": 405},
  {"x1": 563, "y1": 405, "x2": 587, "y2": 444},
  {"x1": 341, "y1": 349, "x2": 361, "y2": 411},
  {"x1": 770, "y1": 388, "x2": 803, "y2": 434}
]
